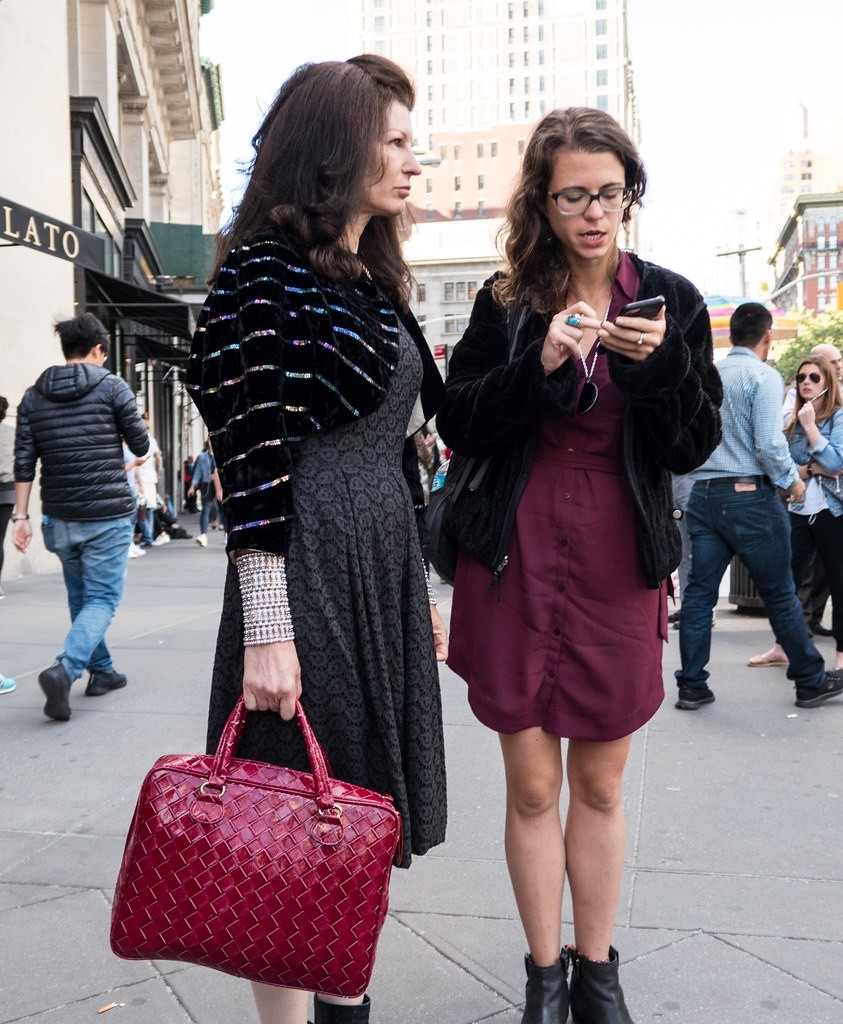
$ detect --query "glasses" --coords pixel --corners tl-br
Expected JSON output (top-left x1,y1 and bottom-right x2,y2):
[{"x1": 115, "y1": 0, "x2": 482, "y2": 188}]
[
  {"x1": 100, "y1": 349, "x2": 109, "y2": 361},
  {"x1": 542, "y1": 187, "x2": 635, "y2": 216},
  {"x1": 796, "y1": 373, "x2": 824, "y2": 383}
]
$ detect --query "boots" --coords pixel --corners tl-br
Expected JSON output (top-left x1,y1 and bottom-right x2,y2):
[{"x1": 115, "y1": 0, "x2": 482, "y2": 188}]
[
  {"x1": 569, "y1": 945, "x2": 634, "y2": 1024},
  {"x1": 520, "y1": 951, "x2": 570, "y2": 1024},
  {"x1": 314, "y1": 994, "x2": 371, "y2": 1024}
]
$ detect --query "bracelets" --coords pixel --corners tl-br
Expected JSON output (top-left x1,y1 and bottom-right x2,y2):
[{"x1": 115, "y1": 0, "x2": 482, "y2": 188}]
[{"x1": 807, "y1": 463, "x2": 815, "y2": 477}]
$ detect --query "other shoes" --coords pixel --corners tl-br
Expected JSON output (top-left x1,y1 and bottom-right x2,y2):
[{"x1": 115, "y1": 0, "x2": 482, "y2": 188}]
[
  {"x1": 674, "y1": 622, "x2": 680, "y2": 630},
  {"x1": 151, "y1": 533, "x2": 170, "y2": 546},
  {"x1": 128, "y1": 544, "x2": 146, "y2": 559},
  {"x1": 195, "y1": 534, "x2": 208, "y2": 547},
  {"x1": 667, "y1": 610, "x2": 680, "y2": 622},
  {"x1": 0, "y1": 674, "x2": 16, "y2": 693},
  {"x1": 811, "y1": 622, "x2": 833, "y2": 637}
]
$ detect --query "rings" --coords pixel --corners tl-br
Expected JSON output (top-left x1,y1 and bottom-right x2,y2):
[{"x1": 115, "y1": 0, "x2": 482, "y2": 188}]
[
  {"x1": 635, "y1": 332, "x2": 646, "y2": 345},
  {"x1": 565, "y1": 314, "x2": 582, "y2": 328}
]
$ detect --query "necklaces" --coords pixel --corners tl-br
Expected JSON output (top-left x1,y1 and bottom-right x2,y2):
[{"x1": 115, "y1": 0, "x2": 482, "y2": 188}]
[{"x1": 566, "y1": 288, "x2": 611, "y2": 415}]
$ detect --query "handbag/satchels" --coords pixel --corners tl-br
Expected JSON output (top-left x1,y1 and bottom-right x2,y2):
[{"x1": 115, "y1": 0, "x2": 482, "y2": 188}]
[
  {"x1": 426, "y1": 280, "x2": 529, "y2": 586},
  {"x1": 111, "y1": 694, "x2": 403, "y2": 997}
]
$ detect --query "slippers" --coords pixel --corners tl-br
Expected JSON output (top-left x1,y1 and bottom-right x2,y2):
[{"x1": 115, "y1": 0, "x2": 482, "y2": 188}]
[{"x1": 747, "y1": 653, "x2": 790, "y2": 667}]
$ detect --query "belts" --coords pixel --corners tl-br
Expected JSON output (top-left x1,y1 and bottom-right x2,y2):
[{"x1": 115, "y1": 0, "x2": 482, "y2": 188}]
[{"x1": 696, "y1": 475, "x2": 769, "y2": 485}]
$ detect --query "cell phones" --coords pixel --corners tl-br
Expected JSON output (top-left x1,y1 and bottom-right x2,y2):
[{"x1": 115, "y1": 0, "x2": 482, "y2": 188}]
[{"x1": 597, "y1": 297, "x2": 666, "y2": 354}]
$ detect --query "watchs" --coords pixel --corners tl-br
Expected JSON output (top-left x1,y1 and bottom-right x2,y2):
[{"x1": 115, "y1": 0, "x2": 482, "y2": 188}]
[{"x1": 11, "y1": 514, "x2": 30, "y2": 523}]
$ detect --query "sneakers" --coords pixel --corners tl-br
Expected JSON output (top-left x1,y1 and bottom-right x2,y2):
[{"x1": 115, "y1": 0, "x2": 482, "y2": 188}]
[
  {"x1": 40, "y1": 663, "x2": 71, "y2": 720},
  {"x1": 795, "y1": 668, "x2": 843, "y2": 708},
  {"x1": 86, "y1": 669, "x2": 126, "y2": 696},
  {"x1": 676, "y1": 680, "x2": 714, "y2": 709}
]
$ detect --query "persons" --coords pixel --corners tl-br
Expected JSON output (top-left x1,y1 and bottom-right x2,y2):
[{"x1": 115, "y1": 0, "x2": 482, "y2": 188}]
[
  {"x1": 0, "y1": 313, "x2": 150, "y2": 722},
  {"x1": 436, "y1": 108, "x2": 724, "y2": 1024},
  {"x1": 415, "y1": 302, "x2": 843, "y2": 711},
  {"x1": 187, "y1": 54, "x2": 450, "y2": 1024},
  {"x1": 122, "y1": 411, "x2": 227, "y2": 560}
]
